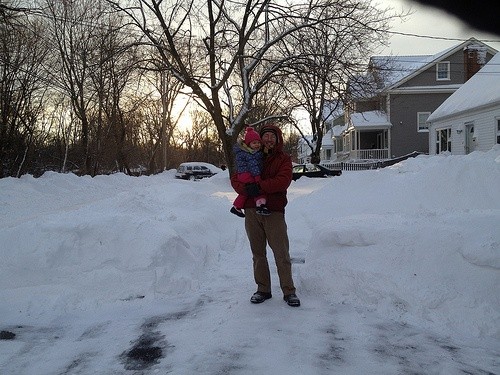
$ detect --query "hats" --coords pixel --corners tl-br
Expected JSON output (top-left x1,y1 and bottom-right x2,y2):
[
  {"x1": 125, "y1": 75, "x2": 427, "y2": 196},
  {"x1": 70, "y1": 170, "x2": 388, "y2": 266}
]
[
  {"x1": 244, "y1": 127, "x2": 261, "y2": 145},
  {"x1": 260, "y1": 128, "x2": 277, "y2": 137}
]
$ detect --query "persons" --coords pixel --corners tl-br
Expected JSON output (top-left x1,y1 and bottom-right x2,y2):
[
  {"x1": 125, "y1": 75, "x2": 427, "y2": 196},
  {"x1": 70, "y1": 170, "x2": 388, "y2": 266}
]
[
  {"x1": 230, "y1": 127, "x2": 271, "y2": 217},
  {"x1": 231, "y1": 123, "x2": 300, "y2": 306}
]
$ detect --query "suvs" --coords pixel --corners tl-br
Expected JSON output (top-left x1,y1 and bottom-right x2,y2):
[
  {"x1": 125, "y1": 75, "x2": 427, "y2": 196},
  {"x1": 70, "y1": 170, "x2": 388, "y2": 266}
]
[{"x1": 174, "y1": 161, "x2": 223, "y2": 181}]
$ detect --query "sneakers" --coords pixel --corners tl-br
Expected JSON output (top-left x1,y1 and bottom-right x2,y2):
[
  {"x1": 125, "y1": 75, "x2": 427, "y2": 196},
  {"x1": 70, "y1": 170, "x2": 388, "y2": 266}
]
[
  {"x1": 256, "y1": 204, "x2": 272, "y2": 215},
  {"x1": 284, "y1": 293, "x2": 300, "y2": 306},
  {"x1": 250, "y1": 291, "x2": 272, "y2": 303},
  {"x1": 230, "y1": 206, "x2": 245, "y2": 217}
]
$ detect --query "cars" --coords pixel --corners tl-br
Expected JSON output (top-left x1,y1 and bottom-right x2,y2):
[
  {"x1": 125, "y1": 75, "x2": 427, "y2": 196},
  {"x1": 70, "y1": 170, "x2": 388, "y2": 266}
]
[{"x1": 291, "y1": 163, "x2": 343, "y2": 181}]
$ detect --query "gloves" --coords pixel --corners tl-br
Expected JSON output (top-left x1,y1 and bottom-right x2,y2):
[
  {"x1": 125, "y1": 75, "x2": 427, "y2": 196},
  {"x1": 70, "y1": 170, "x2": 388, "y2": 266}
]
[
  {"x1": 255, "y1": 176, "x2": 261, "y2": 185},
  {"x1": 245, "y1": 183, "x2": 261, "y2": 195}
]
